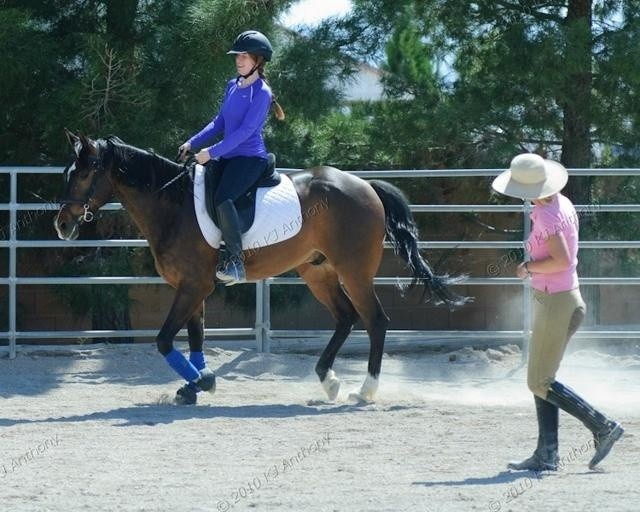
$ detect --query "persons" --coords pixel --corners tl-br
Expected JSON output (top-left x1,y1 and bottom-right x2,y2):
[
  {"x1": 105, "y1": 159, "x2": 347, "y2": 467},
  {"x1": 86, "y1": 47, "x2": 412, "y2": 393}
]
[
  {"x1": 492, "y1": 153, "x2": 624, "y2": 471},
  {"x1": 176, "y1": 31, "x2": 274, "y2": 284}
]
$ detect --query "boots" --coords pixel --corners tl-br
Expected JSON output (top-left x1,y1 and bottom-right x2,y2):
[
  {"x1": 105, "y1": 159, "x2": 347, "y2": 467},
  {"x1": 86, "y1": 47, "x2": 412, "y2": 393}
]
[
  {"x1": 505, "y1": 389, "x2": 559, "y2": 472},
  {"x1": 216, "y1": 196, "x2": 247, "y2": 286},
  {"x1": 542, "y1": 381, "x2": 625, "y2": 470}
]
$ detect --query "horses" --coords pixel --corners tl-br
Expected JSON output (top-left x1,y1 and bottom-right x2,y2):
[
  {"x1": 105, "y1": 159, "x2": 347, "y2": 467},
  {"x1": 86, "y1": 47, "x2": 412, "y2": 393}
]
[{"x1": 52, "y1": 126, "x2": 477, "y2": 406}]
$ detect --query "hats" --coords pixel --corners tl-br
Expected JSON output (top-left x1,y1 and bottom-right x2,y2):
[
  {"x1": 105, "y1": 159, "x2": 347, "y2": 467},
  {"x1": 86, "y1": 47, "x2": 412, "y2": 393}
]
[{"x1": 489, "y1": 152, "x2": 571, "y2": 203}]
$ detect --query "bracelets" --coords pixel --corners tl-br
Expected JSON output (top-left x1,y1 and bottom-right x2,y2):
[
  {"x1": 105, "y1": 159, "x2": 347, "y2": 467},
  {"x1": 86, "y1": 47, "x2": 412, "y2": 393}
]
[{"x1": 523, "y1": 261, "x2": 529, "y2": 274}]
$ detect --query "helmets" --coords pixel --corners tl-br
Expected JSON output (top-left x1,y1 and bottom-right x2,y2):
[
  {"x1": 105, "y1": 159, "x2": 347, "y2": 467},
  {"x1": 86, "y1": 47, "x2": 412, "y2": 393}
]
[{"x1": 225, "y1": 29, "x2": 274, "y2": 63}]
